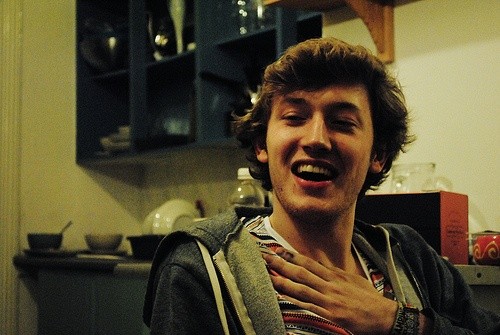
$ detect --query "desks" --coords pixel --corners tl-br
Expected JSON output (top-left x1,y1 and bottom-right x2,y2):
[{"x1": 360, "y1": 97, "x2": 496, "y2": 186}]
[{"x1": 13, "y1": 249, "x2": 500, "y2": 335}]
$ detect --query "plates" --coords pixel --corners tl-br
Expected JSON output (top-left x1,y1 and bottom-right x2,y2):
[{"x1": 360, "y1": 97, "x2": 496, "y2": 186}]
[
  {"x1": 99, "y1": 137, "x2": 130, "y2": 151},
  {"x1": 153, "y1": 199, "x2": 201, "y2": 236},
  {"x1": 142, "y1": 208, "x2": 156, "y2": 236}
]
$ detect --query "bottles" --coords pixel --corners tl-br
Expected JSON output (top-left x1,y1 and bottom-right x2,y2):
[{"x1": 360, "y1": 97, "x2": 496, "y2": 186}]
[
  {"x1": 229, "y1": 167, "x2": 265, "y2": 210},
  {"x1": 391, "y1": 163, "x2": 435, "y2": 192}
]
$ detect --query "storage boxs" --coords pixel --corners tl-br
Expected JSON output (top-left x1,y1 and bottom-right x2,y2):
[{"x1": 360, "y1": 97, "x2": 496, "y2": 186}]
[{"x1": 363, "y1": 191, "x2": 470, "y2": 265}]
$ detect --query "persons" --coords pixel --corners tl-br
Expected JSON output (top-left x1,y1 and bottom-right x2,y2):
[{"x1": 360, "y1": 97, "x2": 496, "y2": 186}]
[{"x1": 144, "y1": 36, "x2": 480, "y2": 335}]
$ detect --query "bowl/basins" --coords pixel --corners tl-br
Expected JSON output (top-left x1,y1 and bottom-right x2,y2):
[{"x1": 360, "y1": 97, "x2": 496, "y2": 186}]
[
  {"x1": 118, "y1": 126, "x2": 129, "y2": 135},
  {"x1": 27, "y1": 233, "x2": 62, "y2": 249},
  {"x1": 125, "y1": 236, "x2": 164, "y2": 259},
  {"x1": 85, "y1": 234, "x2": 122, "y2": 250}
]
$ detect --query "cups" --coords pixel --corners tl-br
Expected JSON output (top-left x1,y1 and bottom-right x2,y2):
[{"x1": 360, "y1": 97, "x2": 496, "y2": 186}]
[{"x1": 471, "y1": 232, "x2": 500, "y2": 264}]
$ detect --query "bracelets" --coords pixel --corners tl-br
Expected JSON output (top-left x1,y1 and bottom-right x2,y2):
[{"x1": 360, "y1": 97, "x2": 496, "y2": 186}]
[{"x1": 392, "y1": 306, "x2": 419, "y2": 335}]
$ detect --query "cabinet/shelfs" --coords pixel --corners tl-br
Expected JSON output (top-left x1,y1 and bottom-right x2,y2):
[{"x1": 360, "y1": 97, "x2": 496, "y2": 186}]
[{"x1": 75, "y1": 0, "x2": 321, "y2": 172}]
